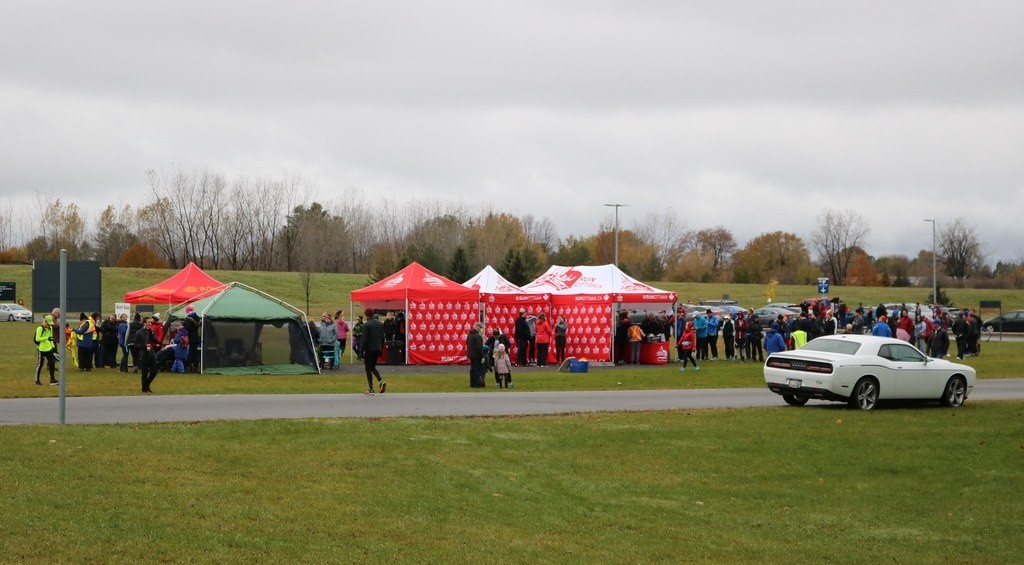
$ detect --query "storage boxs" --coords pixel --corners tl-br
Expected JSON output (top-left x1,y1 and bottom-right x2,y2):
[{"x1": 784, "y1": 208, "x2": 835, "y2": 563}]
[{"x1": 558, "y1": 357, "x2": 589, "y2": 373}]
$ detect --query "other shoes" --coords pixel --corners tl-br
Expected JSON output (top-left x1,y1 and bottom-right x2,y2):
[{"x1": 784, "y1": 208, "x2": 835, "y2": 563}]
[
  {"x1": 142, "y1": 388, "x2": 152, "y2": 394},
  {"x1": 944, "y1": 349, "x2": 980, "y2": 361},
  {"x1": 50, "y1": 380, "x2": 59, "y2": 386},
  {"x1": 468, "y1": 359, "x2": 566, "y2": 389},
  {"x1": 615, "y1": 352, "x2": 765, "y2": 373}
]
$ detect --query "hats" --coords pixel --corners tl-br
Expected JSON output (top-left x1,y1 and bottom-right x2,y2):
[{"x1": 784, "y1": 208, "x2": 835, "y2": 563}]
[
  {"x1": 483, "y1": 345, "x2": 489, "y2": 351},
  {"x1": 475, "y1": 323, "x2": 483, "y2": 328},
  {"x1": 80, "y1": 312, "x2": 88, "y2": 321},
  {"x1": 323, "y1": 312, "x2": 326, "y2": 317},
  {"x1": 498, "y1": 344, "x2": 505, "y2": 352},
  {"x1": 357, "y1": 316, "x2": 362, "y2": 322},
  {"x1": 881, "y1": 316, "x2": 886, "y2": 322},
  {"x1": 826, "y1": 310, "x2": 832, "y2": 315},
  {"x1": 677, "y1": 307, "x2": 790, "y2": 330},
  {"x1": 45, "y1": 316, "x2": 54, "y2": 326},
  {"x1": 519, "y1": 308, "x2": 527, "y2": 312},
  {"x1": 152, "y1": 313, "x2": 160, "y2": 320},
  {"x1": 186, "y1": 305, "x2": 193, "y2": 313},
  {"x1": 931, "y1": 319, "x2": 940, "y2": 324},
  {"x1": 365, "y1": 308, "x2": 374, "y2": 317},
  {"x1": 326, "y1": 315, "x2": 331, "y2": 320}
]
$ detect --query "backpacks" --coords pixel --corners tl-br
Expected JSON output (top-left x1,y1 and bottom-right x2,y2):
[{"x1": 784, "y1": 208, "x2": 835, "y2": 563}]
[
  {"x1": 178, "y1": 333, "x2": 189, "y2": 348},
  {"x1": 34, "y1": 325, "x2": 51, "y2": 345}
]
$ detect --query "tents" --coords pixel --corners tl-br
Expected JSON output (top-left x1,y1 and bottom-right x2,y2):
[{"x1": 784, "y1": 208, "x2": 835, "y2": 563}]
[
  {"x1": 520, "y1": 264, "x2": 678, "y2": 363},
  {"x1": 123, "y1": 262, "x2": 322, "y2": 375},
  {"x1": 350, "y1": 262, "x2": 552, "y2": 364}
]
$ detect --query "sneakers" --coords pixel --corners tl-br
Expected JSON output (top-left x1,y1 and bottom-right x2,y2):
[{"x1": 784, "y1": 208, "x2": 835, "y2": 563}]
[{"x1": 366, "y1": 381, "x2": 386, "y2": 396}]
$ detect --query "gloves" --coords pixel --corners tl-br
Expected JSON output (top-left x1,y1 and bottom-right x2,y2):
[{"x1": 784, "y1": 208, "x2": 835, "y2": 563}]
[{"x1": 49, "y1": 337, "x2": 53, "y2": 341}]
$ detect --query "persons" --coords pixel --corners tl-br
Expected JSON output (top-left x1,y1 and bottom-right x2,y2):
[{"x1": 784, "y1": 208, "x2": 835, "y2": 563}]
[
  {"x1": 467, "y1": 323, "x2": 513, "y2": 389},
  {"x1": 677, "y1": 303, "x2": 687, "y2": 319},
  {"x1": 309, "y1": 310, "x2": 350, "y2": 369},
  {"x1": 617, "y1": 312, "x2": 671, "y2": 366},
  {"x1": 675, "y1": 322, "x2": 700, "y2": 371},
  {"x1": 844, "y1": 303, "x2": 984, "y2": 360},
  {"x1": 35, "y1": 307, "x2": 203, "y2": 393},
  {"x1": 514, "y1": 307, "x2": 567, "y2": 369},
  {"x1": 352, "y1": 307, "x2": 406, "y2": 394},
  {"x1": 677, "y1": 310, "x2": 686, "y2": 362},
  {"x1": 693, "y1": 308, "x2": 764, "y2": 363},
  {"x1": 763, "y1": 297, "x2": 846, "y2": 356},
  {"x1": 352, "y1": 316, "x2": 364, "y2": 361}
]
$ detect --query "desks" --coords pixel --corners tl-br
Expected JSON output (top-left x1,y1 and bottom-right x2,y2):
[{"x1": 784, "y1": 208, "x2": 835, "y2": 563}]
[
  {"x1": 623, "y1": 341, "x2": 669, "y2": 364},
  {"x1": 374, "y1": 341, "x2": 405, "y2": 363}
]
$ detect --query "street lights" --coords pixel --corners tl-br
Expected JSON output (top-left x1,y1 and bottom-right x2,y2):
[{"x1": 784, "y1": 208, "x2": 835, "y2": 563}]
[
  {"x1": 924, "y1": 219, "x2": 936, "y2": 303},
  {"x1": 604, "y1": 203, "x2": 630, "y2": 268}
]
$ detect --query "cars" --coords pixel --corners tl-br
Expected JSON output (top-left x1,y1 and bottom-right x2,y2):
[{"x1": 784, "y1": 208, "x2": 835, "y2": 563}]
[
  {"x1": 0, "y1": 303, "x2": 32, "y2": 323},
  {"x1": 982, "y1": 309, "x2": 1024, "y2": 333},
  {"x1": 763, "y1": 334, "x2": 977, "y2": 413},
  {"x1": 682, "y1": 297, "x2": 966, "y2": 329}
]
